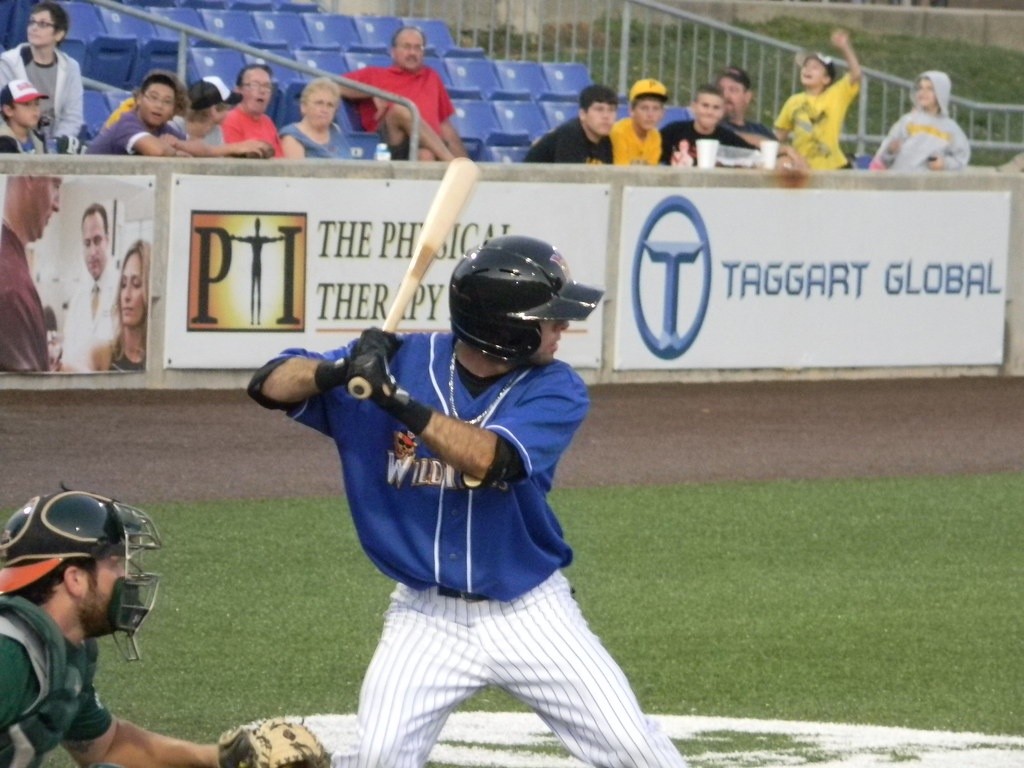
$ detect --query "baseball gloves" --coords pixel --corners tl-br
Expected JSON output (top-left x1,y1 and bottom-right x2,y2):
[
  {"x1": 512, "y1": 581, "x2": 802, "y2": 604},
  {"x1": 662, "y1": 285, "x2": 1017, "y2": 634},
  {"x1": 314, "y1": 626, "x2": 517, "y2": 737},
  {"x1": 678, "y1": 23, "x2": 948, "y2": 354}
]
[{"x1": 216, "y1": 715, "x2": 332, "y2": 768}]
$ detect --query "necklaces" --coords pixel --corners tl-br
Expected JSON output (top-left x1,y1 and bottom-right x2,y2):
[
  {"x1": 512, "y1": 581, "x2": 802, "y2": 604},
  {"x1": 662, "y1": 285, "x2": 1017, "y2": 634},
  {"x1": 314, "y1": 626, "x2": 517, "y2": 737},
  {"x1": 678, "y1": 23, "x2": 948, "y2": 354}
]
[{"x1": 448, "y1": 349, "x2": 518, "y2": 426}]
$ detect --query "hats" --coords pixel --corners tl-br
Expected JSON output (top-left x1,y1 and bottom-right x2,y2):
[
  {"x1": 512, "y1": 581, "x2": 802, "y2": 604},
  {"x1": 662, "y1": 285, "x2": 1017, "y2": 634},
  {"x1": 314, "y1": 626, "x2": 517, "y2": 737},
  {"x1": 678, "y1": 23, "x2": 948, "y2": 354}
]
[
  {"x1": 628, "y1": 77, "x2": 669, "y2": 104},
  {"x1": 0, "y1": 78, "x2": 50, "y2": 105},
  {"x1": 713, "y1": 64, "x2": 752, "y2": 90},
  {"x1": 795, "y1": 48, "x2": 837, "y2": 82},
  {"x1": 187, "y1": 76, "x2": 244, "y2": 111}
]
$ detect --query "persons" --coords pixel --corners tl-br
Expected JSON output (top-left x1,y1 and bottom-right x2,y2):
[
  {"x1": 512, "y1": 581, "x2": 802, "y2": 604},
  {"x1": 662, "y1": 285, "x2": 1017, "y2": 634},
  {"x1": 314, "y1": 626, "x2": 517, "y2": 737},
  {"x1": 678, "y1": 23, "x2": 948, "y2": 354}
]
[
  {"x1": 278, "y1": 77, "x2": 353, "y2": 158},
  {"x1": 0, "y1": 79, "x2": 49, "y2": 155},
  {"x1": 0, "y1": 176, "x2": 71, "y2": 373},
  {"x1": 869, "y1": 71, "x2": 971, "y2": 171},
  {"x1": 521, "y1": 83, "x2": 618, "y2": 164},
  {"x1": 657, "y1": 84, "x2": 757, "y2": 169},
  {"x1": 88, "y1": 239, "x2": 149, "y2": 372},
  {"x1": 86, "y1": 63, "x2": 286, "y2": 158},
  {"x1": 716, "y1": 66, "x2": 808, "y2": 169},
  {"x1": 62, "y1": 201, "x2": 121, "y2": 373},
  {"x1": 0, "y1": 488, "x2": 330, "y2": 768},
  {"x1": 338, "y1": 26, "x2": 471, "y2": 161},
  {"x1": 0, "y1": 2, "x2": 85, "y2": 155},
  {"x1": 246, "y1": 234, "x2": 687, "y2": 768},
  {"x1": 772, "y1": 28, "x2": 863, "y2": 170},
  {"x1": 610, "y1": 78, "x2": 667, "y2": 166}
]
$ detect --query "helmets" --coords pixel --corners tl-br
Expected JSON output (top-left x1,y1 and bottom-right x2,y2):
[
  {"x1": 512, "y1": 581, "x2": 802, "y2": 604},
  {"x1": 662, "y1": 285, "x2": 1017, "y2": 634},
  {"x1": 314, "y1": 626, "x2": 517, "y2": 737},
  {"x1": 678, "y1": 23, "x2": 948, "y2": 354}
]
[
  {"x1": 448, "y1": 234, "x2": 607, "y2": 363},
  {"x1": 0, "y1": 486, "x2": 145, "y2": 598}
]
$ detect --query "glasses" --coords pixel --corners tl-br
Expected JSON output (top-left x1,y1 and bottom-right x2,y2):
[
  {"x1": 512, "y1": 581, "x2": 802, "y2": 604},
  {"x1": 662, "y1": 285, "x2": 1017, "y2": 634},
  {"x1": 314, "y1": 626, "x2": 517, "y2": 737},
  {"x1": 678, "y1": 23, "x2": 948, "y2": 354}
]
[
  {"x1": 141, "y1": 92, "x2": 176, "y2": 108},
  {"x1": 242, "y1": 80, "x2": 273, "y2": 92},
  {"x1": 24, "y1": 17, "x2": 62, "y2": 31},
  {"x1": 394, "y1": 41, "x2": 426, "y2": 53}
]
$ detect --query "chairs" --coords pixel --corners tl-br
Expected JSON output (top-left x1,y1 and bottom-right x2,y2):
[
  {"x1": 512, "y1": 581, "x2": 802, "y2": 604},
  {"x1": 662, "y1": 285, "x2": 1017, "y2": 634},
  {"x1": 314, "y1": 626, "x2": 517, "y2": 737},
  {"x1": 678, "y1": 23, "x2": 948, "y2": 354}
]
[{"x1": 0, "y1": 0, "x2": 873, "y2": 171}]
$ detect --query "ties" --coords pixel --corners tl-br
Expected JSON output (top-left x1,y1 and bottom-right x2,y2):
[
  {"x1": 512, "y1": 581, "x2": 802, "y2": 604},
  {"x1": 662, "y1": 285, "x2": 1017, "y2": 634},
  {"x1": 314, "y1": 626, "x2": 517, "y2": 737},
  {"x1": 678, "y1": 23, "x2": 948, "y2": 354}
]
[{"x1": 89, "y1": 283, "x2": 101, "y2": 320}]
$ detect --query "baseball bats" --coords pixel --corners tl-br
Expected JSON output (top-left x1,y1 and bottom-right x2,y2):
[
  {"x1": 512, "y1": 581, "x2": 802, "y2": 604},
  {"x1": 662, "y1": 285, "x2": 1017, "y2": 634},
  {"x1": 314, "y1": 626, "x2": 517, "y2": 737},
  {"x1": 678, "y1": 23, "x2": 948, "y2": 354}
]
[{"x1": 349, "y1": 155, "x2": 482, "y2": 402}]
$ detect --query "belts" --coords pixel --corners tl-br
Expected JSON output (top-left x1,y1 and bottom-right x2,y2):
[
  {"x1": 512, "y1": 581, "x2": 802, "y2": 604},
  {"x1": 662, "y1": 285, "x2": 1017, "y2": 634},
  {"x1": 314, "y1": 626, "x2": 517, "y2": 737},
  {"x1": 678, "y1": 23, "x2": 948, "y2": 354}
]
[{"x1": 437, "y1": 584, "x2": 491, "y2": 603}]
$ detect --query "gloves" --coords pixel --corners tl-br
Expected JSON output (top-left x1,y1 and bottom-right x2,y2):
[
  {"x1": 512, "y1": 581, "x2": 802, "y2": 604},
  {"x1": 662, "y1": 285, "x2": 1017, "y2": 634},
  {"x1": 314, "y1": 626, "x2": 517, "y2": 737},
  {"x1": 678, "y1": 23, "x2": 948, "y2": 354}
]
[
  {"x1": 346, "y1": 350, "x2": 435, "y2": 437},
  {"x1": 313, "y1": 327, "x2": 405, "y2": 393}
]
